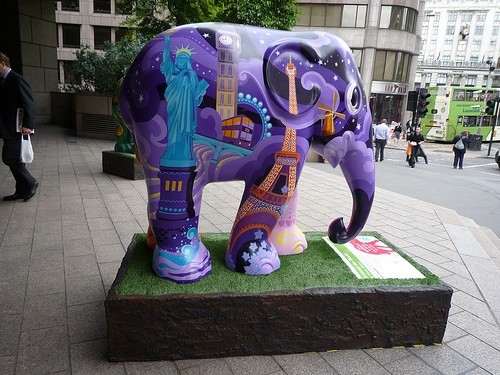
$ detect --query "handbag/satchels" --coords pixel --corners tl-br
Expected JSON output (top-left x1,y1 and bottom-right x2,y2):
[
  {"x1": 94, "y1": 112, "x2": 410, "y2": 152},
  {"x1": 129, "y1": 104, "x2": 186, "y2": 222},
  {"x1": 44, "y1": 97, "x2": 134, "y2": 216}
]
[{"x1": 19, "y1": 131, "x2": 34, "y2": 164}]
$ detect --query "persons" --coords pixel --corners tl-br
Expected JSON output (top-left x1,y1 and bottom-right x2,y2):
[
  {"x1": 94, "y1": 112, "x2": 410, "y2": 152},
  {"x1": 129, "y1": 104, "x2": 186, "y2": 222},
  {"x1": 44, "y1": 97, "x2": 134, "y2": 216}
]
[
  {"x1": 455, "y1": 90, "x2": 500, "y2": 125},
  {"x1": 374, "y1": 116, "x2": 429, "y2": 164},
  {"x1": 452, "y1": 131, "x2": 469, "y2": 170},
  {"x1": 0, "y1": 53, "x2": 39, "y2": 202}
]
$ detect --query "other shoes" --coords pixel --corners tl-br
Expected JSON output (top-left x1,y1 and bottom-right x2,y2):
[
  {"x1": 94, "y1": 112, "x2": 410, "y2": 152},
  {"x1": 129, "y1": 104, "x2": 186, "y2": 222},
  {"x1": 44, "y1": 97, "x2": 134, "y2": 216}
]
[
  {"x1": 380, "y1": 159, "x2": 384, "y2": 161},
  {"x1": 452, "y1": 167, "x2": 457, "y2": 169},
  {"x1": 374, "y1": 160, "x2": 379, "y2": 162},
  {"x1": 394, "y1": 145, "x2": 397, "y2": 146},
  {"x1": 459, "y1": 167, "x2": 463, "y2": 170}
]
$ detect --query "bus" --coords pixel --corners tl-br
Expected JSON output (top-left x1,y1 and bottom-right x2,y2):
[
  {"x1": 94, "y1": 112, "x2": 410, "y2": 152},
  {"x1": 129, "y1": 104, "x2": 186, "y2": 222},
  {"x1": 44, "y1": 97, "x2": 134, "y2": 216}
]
[{"x1": 419, "y1": 85, "x2": 500, "y2": 142}]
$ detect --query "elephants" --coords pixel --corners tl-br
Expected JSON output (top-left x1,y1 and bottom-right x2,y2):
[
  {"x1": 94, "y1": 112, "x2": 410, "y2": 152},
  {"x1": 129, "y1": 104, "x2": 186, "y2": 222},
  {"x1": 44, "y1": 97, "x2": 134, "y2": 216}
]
[{"x1": 120, "y1": 22, "x2": 376, "y2": 284}]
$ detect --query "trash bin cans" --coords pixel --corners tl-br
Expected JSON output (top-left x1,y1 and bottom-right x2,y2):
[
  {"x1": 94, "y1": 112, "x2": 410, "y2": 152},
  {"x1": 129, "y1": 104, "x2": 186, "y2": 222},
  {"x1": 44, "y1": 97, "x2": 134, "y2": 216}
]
[{"x1": 469, "y1": 135, "x2": 483, "y2": 151}]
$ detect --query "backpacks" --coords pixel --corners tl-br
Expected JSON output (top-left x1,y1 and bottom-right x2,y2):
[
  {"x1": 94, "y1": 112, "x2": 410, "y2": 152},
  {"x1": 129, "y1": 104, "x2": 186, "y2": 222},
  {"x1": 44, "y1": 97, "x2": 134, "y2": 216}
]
[{"x1": 455, "y1": 139, "x2": 465, "y2": 149}]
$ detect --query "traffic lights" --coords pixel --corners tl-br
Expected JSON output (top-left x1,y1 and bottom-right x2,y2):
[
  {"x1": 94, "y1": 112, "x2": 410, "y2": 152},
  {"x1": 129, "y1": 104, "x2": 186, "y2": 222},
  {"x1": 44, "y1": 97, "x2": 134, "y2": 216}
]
[
  {"x1": 485, "y1": 96, "x2": 499, "y2": 118},
  {"x1": 415, "y1": 89, "x2": 430, "y2": 119}
]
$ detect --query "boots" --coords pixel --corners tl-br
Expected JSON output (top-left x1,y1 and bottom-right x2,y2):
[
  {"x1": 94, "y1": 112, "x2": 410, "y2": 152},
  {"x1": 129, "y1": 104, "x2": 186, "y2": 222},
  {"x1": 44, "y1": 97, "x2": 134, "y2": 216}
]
[
  {"x1": 424, "y1": 156, "x2": 428, "y2": 164},
  {"x1": 406, "y1": 154, "x2": 410, "y2": 161}
]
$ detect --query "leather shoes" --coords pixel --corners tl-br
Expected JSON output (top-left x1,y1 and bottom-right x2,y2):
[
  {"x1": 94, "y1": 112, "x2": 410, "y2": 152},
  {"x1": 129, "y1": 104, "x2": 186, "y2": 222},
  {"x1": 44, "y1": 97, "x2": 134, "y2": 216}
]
[
  {"x1": 2, "y1": 192, "x2": 26, "y2": 201},
  {"x1": 23, "y1": 181, "x2": 39, "y2": 202}
]
